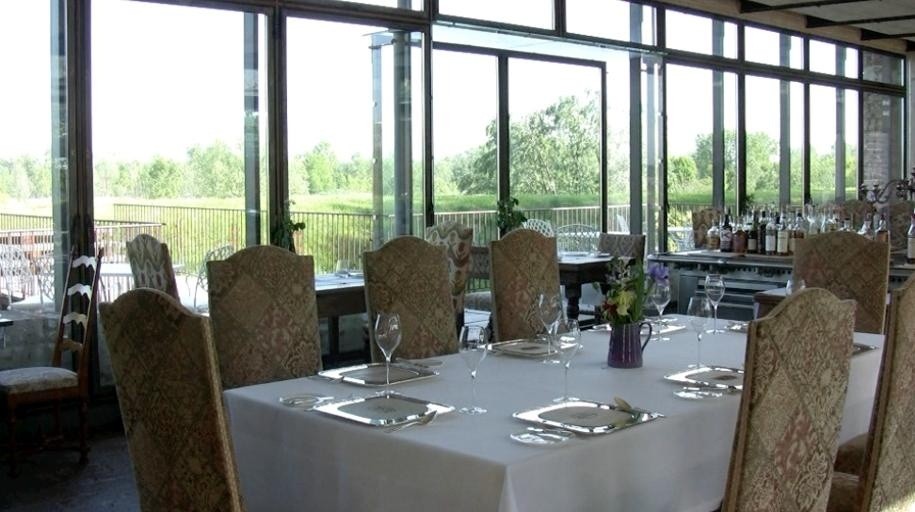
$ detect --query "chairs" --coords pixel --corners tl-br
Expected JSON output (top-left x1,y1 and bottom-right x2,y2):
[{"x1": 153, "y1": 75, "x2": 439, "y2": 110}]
[{"x1": 1, "y1": 241, "x2": 103, "y2": 499}]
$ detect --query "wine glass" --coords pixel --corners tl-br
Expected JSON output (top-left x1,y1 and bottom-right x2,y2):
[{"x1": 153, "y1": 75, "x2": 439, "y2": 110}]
[
  {"x1": 457, "y1": 324, "x2": 490, "y2": 417},
  {"x1": 537, "y1": 291, "x2": 566, "y2": 366},
  {"x1": 335, "y1": 259, "x2": 350, "y2": 286},
  {"x1": 783, "y1": 277, "x2": 808, "y2": 297},
  {"x1": 703, "y1": 273, "x2": 727, "y2": 337},
  {"x1": 649, "y1": 276, "x2": 673, "y2": 343},
  {"x1": 373, "y1": 309, "x2": 408, "y2": 397},
  {"x1": 551, "y1": 318, "x2": 583, "y2": 404},
  {"x1": 686, "y1": 295, "x2": 711, "y2": 370}
]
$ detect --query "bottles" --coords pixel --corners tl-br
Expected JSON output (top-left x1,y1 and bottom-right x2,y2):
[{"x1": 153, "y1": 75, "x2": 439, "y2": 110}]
[
  {"x1": 906, "y1": 213, "x2": 915, "y2": 265},
  {"x1": 705, "y1": 200, "x2": 893, "y2": 258},
  {"x1": 604, "y1": 321, "x2": 654, "y2": 370}
]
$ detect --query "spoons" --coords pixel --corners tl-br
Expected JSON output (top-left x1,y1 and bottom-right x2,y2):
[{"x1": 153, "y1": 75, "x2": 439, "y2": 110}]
[
  {"x1": 612, "y1": 396, "x2": 667, "y2": 418},
  {"x1": 381, "y1": 409, "x2": 438, "y2": 437},
  {"x1": 394, "y1": 354, "x2": 431, "y2": 369},
  {"x1": 474, "y1": 345, "x2": 507, "y2": 357}
]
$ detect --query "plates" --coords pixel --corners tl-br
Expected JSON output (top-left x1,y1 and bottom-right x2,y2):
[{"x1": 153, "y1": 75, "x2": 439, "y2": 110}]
[
  {"x1": 410, "y1": 356, "x2": 446, "y2": 369},
  {"x1": 671, "y1": 385, "x2": 726, "y2": 402},
  {"x1": 544, "y1": 334, "x2": 577, "y2": 350},
  {"x1": 723, "y1": 320, "x2": 752, "y2": 335},
  {"x1": 316, "y1": 359, "x2": 440, "y2": 387},
  {"x1": 594, "y1": 317, "x2": 685, "y2": 338},
  {"x1": 663, "y1": 362, "x2": 744, "y2": 393},
  {"x1": 509, "y1": 426, "x2": 578, "y2": 447},
  {"x1": 850, "y1": 341, "x2": 875, "y2": 357},
  {"x1": 315, "y1": 389, "x2": 456, "y2": 429},
  {"x1": 478, "y1": 335, "x2": 578, "y2": 360},
  {"x1": 510, "y1": 398, "x2": 658, "y2": 436},
  {"x1": 277, "y1": 392, "x2": 326, "y2": 409},
  {"x1": 348, "y1": 272, "x2": 364, "y2": 278}
]
